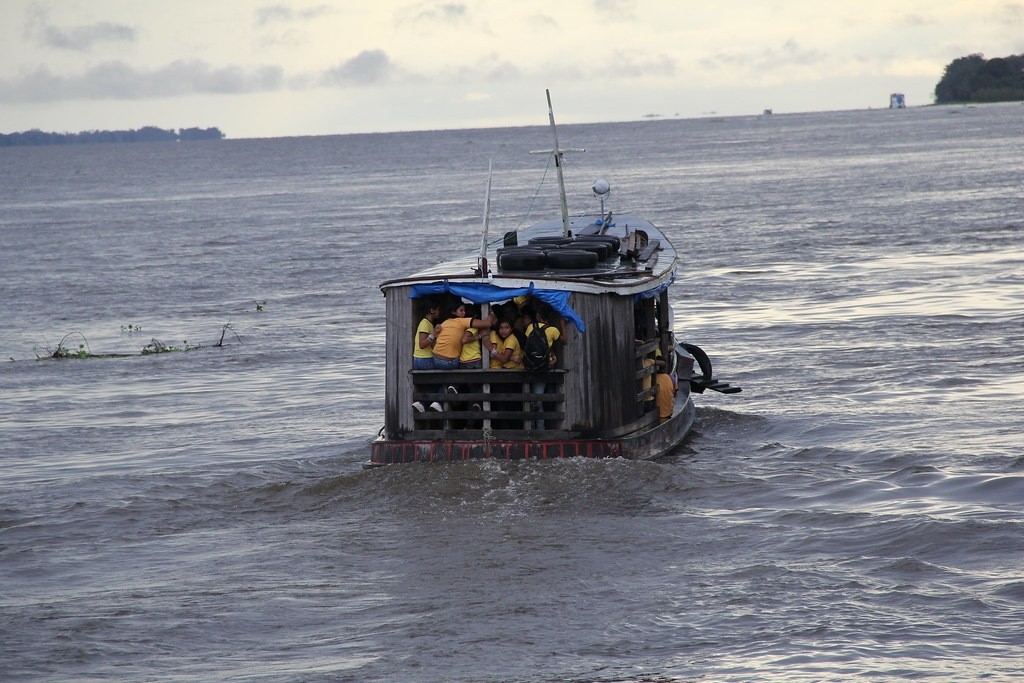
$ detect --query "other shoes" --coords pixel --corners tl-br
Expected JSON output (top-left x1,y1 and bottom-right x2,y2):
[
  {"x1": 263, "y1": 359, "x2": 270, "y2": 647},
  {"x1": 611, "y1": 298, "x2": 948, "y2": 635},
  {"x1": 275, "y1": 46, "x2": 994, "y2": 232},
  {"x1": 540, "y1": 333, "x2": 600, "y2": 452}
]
[
  {"x1": 447, "y1": 384, "x2": 459, "y2": 394},
  {"x1": 411, "y1": 400, "x2": 425, "y2": 412},
  {"x1": 472, "y1": 402, "x2": 483, "y2": 411},
  {"x1": 429, "y1": 401, "x2": 442, "y2": 412}
]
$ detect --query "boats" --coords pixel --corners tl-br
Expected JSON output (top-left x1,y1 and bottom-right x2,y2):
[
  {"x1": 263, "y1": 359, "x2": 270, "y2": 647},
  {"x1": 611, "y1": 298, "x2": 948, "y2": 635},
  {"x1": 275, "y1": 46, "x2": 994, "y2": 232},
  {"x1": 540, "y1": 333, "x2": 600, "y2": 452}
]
[{"x1": 360, "y1": 87, "x2": 743, "y2": 472}]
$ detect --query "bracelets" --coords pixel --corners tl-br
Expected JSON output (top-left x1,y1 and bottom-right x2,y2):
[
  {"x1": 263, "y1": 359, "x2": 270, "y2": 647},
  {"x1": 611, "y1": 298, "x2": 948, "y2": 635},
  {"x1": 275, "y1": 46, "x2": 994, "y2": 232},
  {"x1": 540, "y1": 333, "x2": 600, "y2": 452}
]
[
  {"x1": 426, "y1": 333, "x2": 435, "y2": 343},
  {"x1": 489, "y1": 311, "x2": 495, "y2": 316},
  {"x1": 490, "y1": 348, "x2": 498, "y2": 357}
]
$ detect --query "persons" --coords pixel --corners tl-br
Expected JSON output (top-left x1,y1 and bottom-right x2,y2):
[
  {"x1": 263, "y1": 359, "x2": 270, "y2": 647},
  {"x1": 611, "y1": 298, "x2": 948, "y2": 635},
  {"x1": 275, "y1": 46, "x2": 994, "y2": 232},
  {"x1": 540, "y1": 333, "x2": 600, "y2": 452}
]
[
  {"x1": 429, "y1": 299, "x2": 494, "y2": 429},
  {"x1": 489, "y1": 304, "x2": 506, "y2": 429},
  {"x1": 496, "y1": 301, "x2": 535, "y2": 350},
  {"x1": 482, "y1": 317, "x2": 525, "y2": 430},
  {"x1": 523, "y1": 305, "x2": 568, "y2": 430},
  {"x1": 412, "y1": 302, "x2": 442, "y2": 413},
  {"x1": 636, "y1": 299, "x2": 681, "y2": 419}
]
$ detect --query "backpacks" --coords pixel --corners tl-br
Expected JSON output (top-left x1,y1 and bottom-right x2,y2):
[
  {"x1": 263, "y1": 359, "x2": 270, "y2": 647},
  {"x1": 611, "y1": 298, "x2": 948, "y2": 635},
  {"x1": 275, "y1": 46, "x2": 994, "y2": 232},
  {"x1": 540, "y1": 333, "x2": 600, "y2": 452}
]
[{"x1": 523, "y1": 320, "x2": 551, "y2": 373}]
